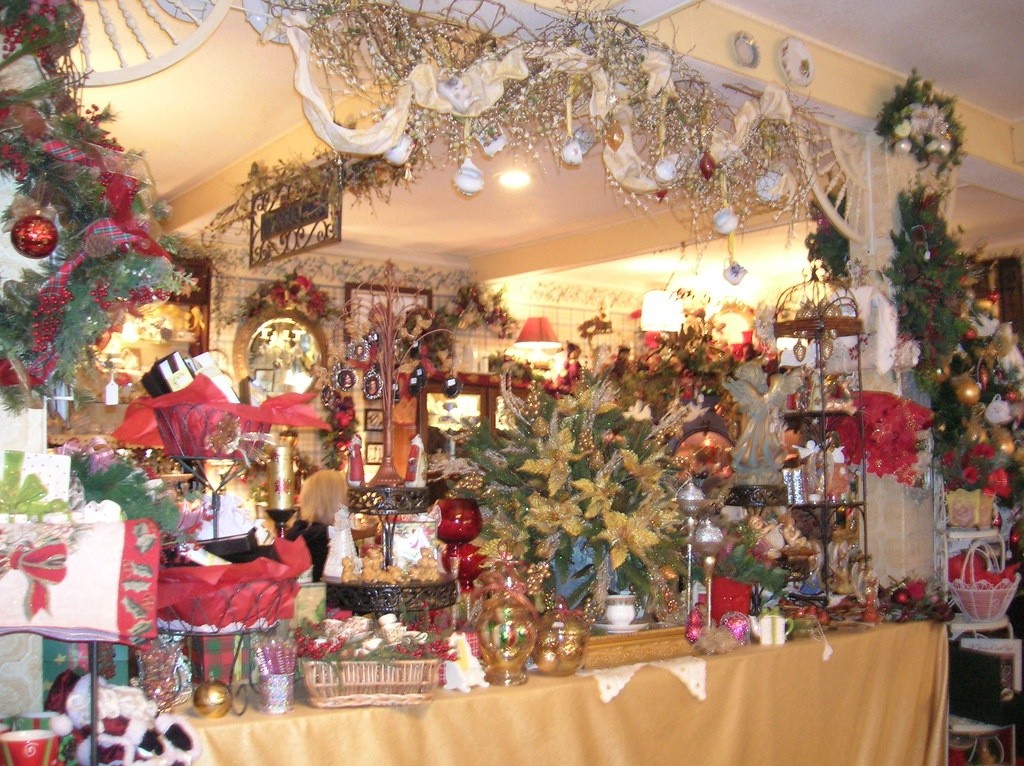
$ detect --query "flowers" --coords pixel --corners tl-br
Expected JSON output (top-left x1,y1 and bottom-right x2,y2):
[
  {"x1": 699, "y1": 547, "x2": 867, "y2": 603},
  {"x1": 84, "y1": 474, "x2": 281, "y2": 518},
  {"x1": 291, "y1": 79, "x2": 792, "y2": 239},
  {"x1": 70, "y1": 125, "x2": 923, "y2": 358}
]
[
  {"x1": 421, "y1": 345, "x2": 710, "y2": 627},
  {"x1": 225, "y1": 258, "x2": 335, "y2": 325}
]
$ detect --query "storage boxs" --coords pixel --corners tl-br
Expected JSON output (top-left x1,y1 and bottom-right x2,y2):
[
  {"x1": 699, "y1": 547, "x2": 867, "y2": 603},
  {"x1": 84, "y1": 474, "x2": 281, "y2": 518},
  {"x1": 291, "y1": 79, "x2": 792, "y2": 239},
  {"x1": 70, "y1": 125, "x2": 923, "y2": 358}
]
[{"x1": 181, "y1": 632, "x2": 252, "y2": 685}]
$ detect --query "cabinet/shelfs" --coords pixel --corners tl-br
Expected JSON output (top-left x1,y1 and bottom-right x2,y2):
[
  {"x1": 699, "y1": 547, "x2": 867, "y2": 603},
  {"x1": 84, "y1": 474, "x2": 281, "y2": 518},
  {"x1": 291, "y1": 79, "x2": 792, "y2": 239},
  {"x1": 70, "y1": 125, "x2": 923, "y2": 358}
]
[
  {"x1": 418, "y1": 382, "x2": 528, "y2": 455},
  {"x1": 946, "y1": 526, "x2": 1017, "y2": 766},
  {"x1": 95, "y1": 256, "x2": 212, "y2": 376},
  {"x1": 772, "y1": 265, "x2": 870, "y2": 632}
]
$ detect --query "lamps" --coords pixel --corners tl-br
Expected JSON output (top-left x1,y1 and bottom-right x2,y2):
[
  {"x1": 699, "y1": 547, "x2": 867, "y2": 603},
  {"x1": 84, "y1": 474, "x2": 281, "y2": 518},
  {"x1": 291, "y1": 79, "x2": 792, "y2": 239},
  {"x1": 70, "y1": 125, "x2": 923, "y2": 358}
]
[{"x1": 513, "y1": 318, "x2": 565, "y2": 350}]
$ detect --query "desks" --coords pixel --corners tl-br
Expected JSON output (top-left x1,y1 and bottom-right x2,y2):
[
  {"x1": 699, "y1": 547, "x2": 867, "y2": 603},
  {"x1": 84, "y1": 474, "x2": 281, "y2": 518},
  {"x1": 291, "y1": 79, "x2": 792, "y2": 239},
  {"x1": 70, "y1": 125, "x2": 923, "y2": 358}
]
[{"x1": 167, "y1": 621, "x2": 947, "y2": 766}]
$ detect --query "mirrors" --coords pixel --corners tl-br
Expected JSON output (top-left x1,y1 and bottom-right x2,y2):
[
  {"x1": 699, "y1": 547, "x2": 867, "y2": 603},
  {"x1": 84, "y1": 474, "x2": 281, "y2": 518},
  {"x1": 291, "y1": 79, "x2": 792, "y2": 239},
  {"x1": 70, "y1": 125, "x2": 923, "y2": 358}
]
[{"x1": 233, "y1": 307, "x2": 330, "y2": 407}]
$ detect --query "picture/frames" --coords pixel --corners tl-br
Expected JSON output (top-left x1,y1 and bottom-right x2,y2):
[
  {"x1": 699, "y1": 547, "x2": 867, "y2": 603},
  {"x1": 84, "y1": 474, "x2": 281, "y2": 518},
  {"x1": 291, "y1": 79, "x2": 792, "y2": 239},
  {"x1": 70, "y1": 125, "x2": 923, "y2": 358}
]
[
  {"x1": 364, "y1": 408, "x2": 383, "y2": 431},
  {"x1": 365, "y1": 442, "x2": 383, "y2": 465},
  {"x1": 344, "y1": 282, "x2": 433, "y2": 344}
]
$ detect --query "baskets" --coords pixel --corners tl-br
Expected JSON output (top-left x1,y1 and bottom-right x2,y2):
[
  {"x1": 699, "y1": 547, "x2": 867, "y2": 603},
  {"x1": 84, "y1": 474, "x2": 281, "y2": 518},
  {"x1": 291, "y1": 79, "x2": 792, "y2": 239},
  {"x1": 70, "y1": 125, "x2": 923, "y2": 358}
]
[
  {"x1": 947, "y1": 534, "x2": 1021, "y2": 623},
  {"x1": 302, "y1": 660, "x2": 441, "y2": 710}
]
